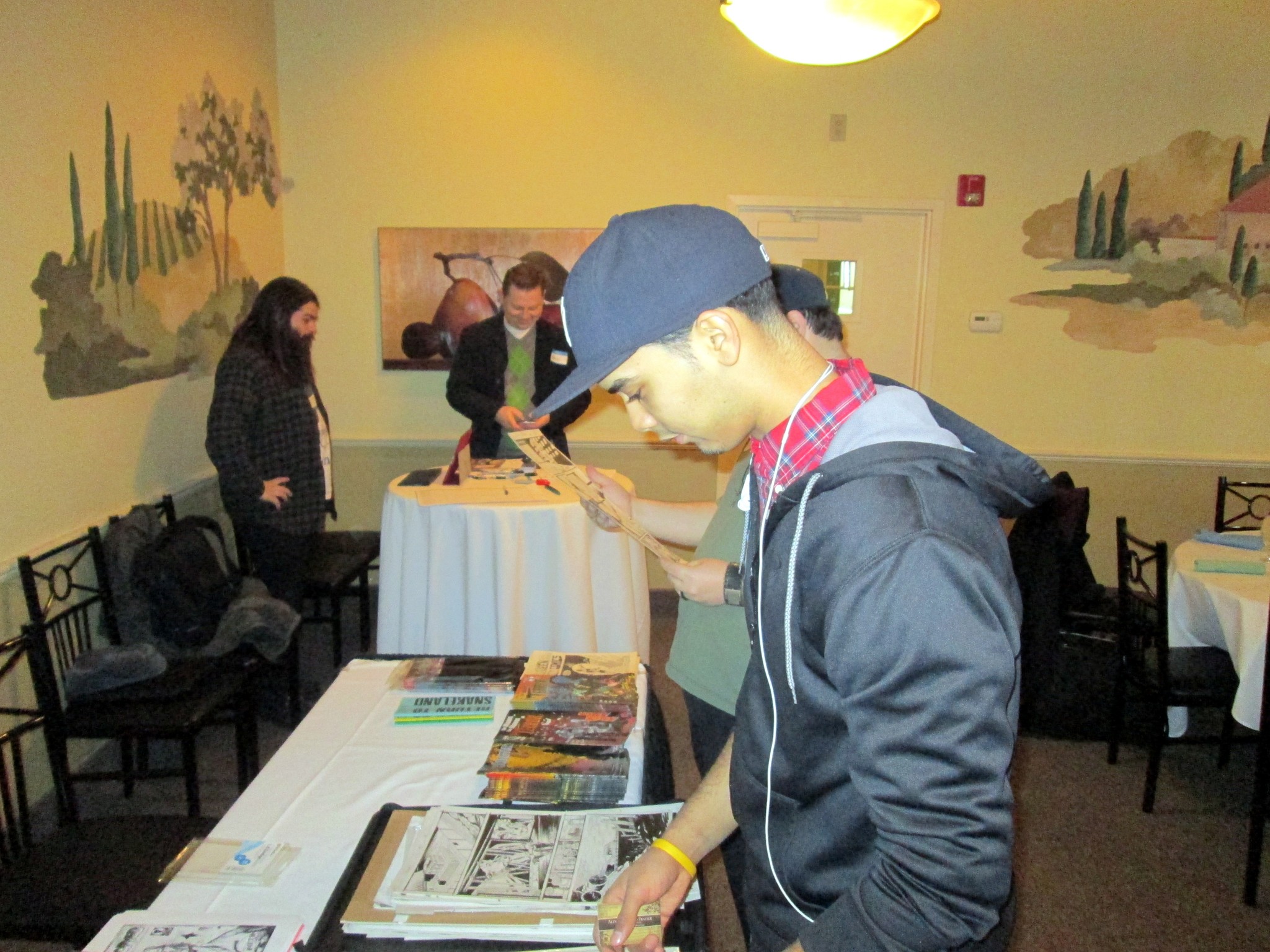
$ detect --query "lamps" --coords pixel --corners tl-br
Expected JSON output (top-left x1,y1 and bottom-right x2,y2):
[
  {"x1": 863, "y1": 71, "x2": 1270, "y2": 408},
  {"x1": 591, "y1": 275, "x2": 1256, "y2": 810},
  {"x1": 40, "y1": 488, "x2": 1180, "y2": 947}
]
[{"x1": 720, "y1": 0, "x2": 941, "y2": 66}]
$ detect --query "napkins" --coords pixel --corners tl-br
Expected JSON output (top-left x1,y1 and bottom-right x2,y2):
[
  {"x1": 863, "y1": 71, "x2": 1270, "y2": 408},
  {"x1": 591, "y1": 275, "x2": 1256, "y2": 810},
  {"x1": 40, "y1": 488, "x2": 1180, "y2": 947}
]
[
  {"x1": 1193, "y1": 558, "x2": 1265, "y2": 575},
  {"x1": 1194, "y1": 526, "x2": 1263, "y2": 551}
]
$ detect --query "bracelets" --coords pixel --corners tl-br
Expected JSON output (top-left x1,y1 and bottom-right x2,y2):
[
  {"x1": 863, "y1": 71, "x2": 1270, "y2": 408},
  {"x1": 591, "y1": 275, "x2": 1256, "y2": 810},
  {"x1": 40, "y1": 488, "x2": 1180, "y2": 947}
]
[{"x1": 651, "y1": 838, "x2": 697, "y2": 879}]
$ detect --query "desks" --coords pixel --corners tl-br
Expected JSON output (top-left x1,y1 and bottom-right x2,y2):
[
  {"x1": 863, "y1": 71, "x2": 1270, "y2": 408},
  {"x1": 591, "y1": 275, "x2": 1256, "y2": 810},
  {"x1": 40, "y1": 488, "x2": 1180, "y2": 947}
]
[
  {"x1": 376, "y1": 464, "x2": 651, "y2": 667},
  {"x1": 1167, "y1": 530, "x2": 1270, "y2": 740},
  {"x1": 141, "y1": 657, "x2": 693, "y2": 952}
]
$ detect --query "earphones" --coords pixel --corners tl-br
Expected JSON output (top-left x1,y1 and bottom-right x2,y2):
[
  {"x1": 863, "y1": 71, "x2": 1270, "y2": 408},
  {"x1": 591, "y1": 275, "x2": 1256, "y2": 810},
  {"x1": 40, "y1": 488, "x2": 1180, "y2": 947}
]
[{"x1": 737, "y1": 474, "x2": 750, "y2": 512}]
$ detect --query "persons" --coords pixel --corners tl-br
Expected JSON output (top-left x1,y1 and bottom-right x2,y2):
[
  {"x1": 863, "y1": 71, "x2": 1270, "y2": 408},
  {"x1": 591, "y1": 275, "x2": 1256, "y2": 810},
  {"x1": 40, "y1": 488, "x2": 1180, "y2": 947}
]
[
  {"x1": 574, "y1": 263, "x2": 859, "y2": 781},
  {"x1": 445, "y1": 264, "x2": 594, "y2": 461},
  {"x1": 526, "y1": 202, "x2": 1054, "y2": 952},
  {"x1": 205, "y1": 274, "x2": 337, "y2": 724}
]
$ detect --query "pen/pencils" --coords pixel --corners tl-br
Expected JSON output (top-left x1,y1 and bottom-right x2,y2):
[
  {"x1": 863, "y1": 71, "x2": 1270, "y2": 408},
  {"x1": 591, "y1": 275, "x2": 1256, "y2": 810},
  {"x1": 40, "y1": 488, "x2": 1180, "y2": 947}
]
[
  {"x1": 511, "y1": 472, "x2": 537, "y2": 477},
  {"x1": 491, "y1": 476, "x2": 513, "y2": 479},
  {"x1": 544, "y1": 485, "x2": 561, "y2": 495}
]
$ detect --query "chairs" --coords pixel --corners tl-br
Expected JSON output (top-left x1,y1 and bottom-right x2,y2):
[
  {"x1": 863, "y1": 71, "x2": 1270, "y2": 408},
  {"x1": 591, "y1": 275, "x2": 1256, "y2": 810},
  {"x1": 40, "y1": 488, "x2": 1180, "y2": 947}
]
[
  {"x1": 19, "y1": 493, "x2": 380, "y2": 822},
  {"x1": 1027, "y1": 469, "x2": 1270, "y2": 906}
]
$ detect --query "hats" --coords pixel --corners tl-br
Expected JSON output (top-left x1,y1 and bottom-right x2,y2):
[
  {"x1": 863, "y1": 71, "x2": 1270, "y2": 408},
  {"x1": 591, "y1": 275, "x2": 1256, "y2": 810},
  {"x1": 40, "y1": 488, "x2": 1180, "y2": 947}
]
[
  {"x1": 531, "y1": 203, "x2": 772, "y2": 420},
  {"x1": 771, "y1": 264, "x2": 828, "y2": 314}
]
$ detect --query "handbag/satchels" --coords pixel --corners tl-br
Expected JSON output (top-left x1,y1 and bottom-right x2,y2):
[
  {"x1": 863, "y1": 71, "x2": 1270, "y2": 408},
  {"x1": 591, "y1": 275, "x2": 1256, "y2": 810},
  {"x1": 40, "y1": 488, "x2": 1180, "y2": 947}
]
[{"x1": 131, "y1": 515, "x2": 242, "y2": 650}]
